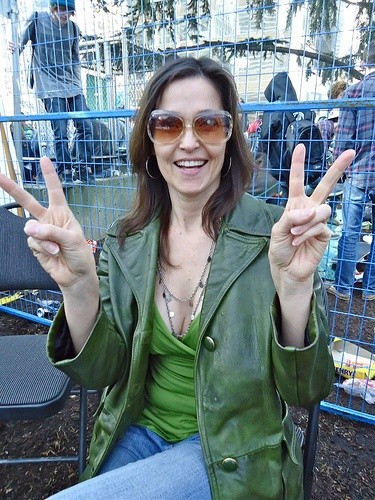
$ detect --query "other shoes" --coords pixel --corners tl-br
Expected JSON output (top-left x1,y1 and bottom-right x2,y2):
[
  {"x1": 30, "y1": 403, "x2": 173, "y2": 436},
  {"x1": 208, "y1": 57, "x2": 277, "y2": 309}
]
[
  {"x1": 73, "y1": 165, "x2": 97, "y2": 185},
  {"x1": 325, "y1": 280, "x2": 350, "y2": 301},
  {"x1": 58, "y1": 169, "x2": 73, "y2": 184},
  {"x1": 362, "y1": 292, "x2": 375, "y2": 301}
]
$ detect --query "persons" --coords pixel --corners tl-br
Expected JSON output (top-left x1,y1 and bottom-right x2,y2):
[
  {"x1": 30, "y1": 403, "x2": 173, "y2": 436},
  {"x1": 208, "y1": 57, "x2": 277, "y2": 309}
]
[
  {"x1": 5, "y1": 42, "x2": 375, "y2": 301},
  {"x1": 0, "y1": 56, "x2": 355, "y2": 500},
  {"x1": 7, "y1": 0, "x2": 95, "y2": 184}
]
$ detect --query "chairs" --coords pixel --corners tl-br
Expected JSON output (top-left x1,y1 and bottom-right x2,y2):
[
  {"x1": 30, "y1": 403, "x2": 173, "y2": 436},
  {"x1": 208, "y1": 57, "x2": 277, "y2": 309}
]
[{"x1": 0, "y1": 202, "x2": 110, "y2": 484}]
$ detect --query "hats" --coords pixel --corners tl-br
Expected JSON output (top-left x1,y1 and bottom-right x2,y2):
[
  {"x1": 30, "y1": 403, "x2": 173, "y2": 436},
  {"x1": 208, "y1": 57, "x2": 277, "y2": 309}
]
[{"x1": 49, "y1": 0, "x2": 75, "y2": 10}]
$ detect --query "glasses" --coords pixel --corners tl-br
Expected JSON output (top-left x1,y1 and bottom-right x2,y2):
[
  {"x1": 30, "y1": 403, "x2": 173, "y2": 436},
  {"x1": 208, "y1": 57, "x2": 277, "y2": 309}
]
[{"x1": 146, "y1": 109, "x2": 233, "y2": 145}]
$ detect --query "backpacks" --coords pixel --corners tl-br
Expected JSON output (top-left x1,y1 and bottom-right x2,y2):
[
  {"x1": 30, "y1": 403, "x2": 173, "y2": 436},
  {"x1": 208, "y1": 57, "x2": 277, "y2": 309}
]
[
  {"x1": 284, "y1": 109, "x2": 327, "y2": 187},
  {"x1": 69, "y1": 106, "x2": 114, "y2": 170}
]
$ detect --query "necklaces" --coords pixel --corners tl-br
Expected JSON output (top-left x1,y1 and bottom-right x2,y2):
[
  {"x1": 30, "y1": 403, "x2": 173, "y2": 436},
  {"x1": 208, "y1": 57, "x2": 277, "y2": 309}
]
[{"x1": 157, "y1": 235, "x2": 214, "y2": 339}]
